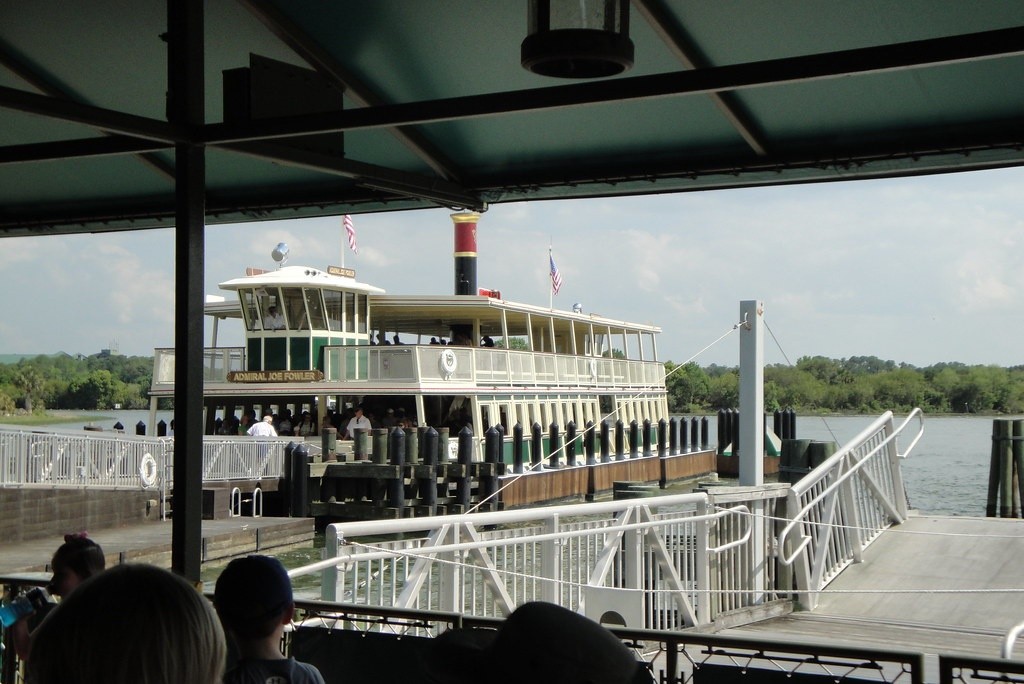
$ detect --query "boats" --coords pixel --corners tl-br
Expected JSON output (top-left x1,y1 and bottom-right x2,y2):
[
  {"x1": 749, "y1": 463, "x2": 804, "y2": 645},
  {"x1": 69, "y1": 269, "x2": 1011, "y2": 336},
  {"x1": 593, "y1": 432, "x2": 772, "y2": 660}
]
[{"x1": 148, "y1": 213, "x2": 670, "y2": 480}]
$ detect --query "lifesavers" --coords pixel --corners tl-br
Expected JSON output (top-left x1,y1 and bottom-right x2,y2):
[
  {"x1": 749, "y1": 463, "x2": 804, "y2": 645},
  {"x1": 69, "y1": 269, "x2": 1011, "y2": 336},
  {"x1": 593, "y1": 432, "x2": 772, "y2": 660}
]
[
  {"x1": 440, "y1": 348, "x2": 458, "y2": 373},
  {"x1": 589, "y1": 359, "x2": 598, "y2": 379},
  {"x1": 139, "y1": 453, "x2": 157, "y2": 486}
]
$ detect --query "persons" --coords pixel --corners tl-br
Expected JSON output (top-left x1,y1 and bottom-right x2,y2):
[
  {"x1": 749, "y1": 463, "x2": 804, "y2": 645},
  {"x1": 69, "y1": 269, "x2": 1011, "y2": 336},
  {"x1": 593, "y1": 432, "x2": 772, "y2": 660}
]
[
  {"x1": 420, "y1": 601, "x2": 653, "y2": 684},
  {"x1": 12, "y1": 536, "x2": 106, "y2": 660},
  {"x1": 25, "y1": 564, "x2": 227, "y2": 684},
  {"x1": 213, "y1": 555, "x2": 324, "y2": 684},
  {"x1": 263, "y1": 306, "x2": 285, "y2": 329},
  {"x1": 376, "y1": 334, "x2": 391, "y2": 344},
  {"x1": 367, "y1": 408, "x2": 417, "y2": 433},
  {"x1": 343, "y1": 407, "x2": 372, "y2": 440},
  {"x1": 370, "y1": 334, "x2": 376, "y2": 345},
  {"x1": 430, "y1": 337, "x2": 440, "y2": 344},
  {"x1": 462, "y1": 415, "x2": 472, "y2": 428},
  {"x1": 218, "y1": 409, "x2": 352, "y2": 440},
  {"x1": 394, "y1": 336, "x2": 404, "y2": 344},
  {"x1": 441, "y1": 340, "x2": 446, "y2": 345},
  {"x1": 448, "y1": 342, "x2": 453, "y2": 345},
  {"x1": 480, "y1": 336, "x2": 494, "y2": 347}
]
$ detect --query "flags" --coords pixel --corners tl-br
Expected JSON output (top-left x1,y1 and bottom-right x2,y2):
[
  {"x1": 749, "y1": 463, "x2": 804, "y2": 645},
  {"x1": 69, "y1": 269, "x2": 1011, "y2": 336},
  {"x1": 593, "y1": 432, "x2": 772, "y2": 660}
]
[
  {"x1": 550, "y1": 255, "x2": 562, "y2": 295},
  {"x1": 344, "y1": 214, "x2": 358, "y2": 253}
]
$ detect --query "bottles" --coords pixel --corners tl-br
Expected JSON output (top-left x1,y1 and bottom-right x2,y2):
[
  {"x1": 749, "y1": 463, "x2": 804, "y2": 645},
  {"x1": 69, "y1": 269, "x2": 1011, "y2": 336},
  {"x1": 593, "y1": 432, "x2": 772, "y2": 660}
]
[{"x1": 0, "y1": 586, "x2": 52, "y2": 627}]
